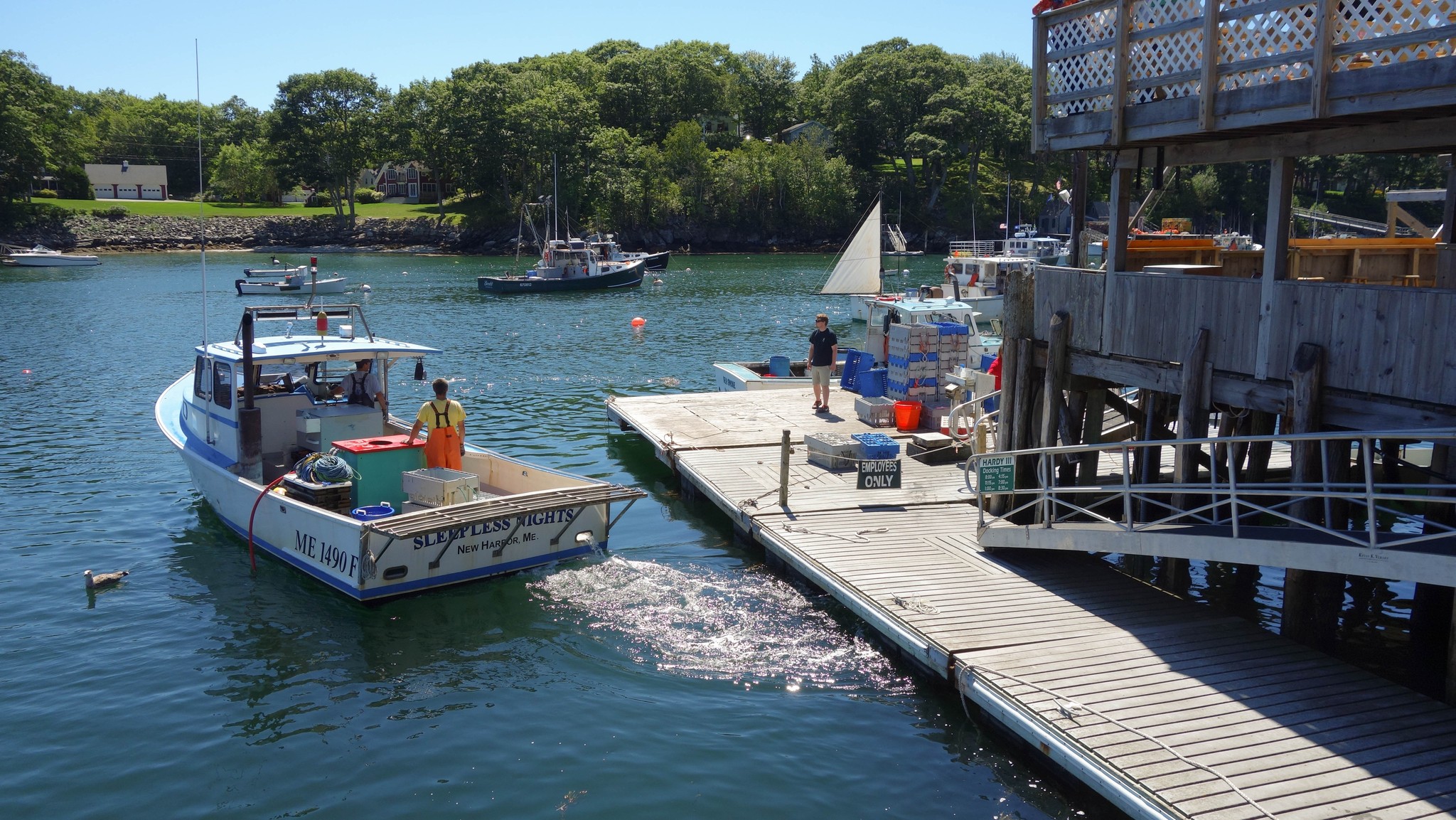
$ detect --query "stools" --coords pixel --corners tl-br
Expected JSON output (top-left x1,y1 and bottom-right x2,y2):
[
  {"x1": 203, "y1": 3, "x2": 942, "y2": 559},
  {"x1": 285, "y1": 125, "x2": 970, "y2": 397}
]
[
  {"x1": 1298, "y1": 277, "x2": 1325, "y2": 282},
  {"x1": 1341, "y1": 275, "x2": 1369, "y2": 284},
  {"x1": 1391, "y1": 275, "x2": 1420, "y2": 287}
]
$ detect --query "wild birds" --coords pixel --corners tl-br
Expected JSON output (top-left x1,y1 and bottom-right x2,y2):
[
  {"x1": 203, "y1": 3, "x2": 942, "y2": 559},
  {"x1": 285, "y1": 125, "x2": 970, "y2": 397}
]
[{"x1": 83, "y1": 570, "x2": 129, "y2": 588}]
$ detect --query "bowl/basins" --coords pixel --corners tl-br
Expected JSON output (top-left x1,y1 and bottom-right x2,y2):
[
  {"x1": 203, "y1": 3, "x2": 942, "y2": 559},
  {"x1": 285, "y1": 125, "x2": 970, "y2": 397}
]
[{"x1": 763, "y1": 374, "x2": 777, "y2": 377}]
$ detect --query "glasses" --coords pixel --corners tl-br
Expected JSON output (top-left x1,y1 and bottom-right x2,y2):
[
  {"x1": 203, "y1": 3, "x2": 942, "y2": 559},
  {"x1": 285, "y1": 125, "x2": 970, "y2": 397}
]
[{"x1": 815, "y1": 319, "x2": 826, "y2": 322}]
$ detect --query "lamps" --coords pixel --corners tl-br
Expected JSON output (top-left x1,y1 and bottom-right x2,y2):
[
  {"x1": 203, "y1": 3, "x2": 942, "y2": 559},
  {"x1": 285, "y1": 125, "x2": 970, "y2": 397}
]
[
  {"x1": 973, "y1": 260, "x2": 977, "y2": 263},
  {"x1": 955, "y1": 260, "x2": 959, "y2": 263}
]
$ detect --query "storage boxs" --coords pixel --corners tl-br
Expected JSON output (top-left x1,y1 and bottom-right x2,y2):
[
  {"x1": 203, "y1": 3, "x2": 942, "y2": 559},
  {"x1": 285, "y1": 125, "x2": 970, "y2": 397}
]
[
  {"x1": 283, "y1": 474, "x2": 352, "y2": 518},
  {"x1": 804, "y1": 321, "x2": 998, "y2": 469},
  {"x1": 296, "y1": 416, "x2": 320, "y2": 433},
  {"x1": 527, "y1": 270, "x2": 536, "y2": 279},
  {"x1": 401, "y1": 466, "x2": 480, "y2": 514}
]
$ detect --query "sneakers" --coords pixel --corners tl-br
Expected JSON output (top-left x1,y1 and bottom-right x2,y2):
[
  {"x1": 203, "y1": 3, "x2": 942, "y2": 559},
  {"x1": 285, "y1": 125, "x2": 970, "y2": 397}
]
[
  {"x1": 812, "y1": 399, "x2": 822, "y2": 409},
  {"x1": 817, "y1": 405, "x2": 829, "y2": 413}
]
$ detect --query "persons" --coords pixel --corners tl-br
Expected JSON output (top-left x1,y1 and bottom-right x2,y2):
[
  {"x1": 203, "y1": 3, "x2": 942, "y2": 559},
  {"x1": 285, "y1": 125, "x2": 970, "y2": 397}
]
[
  {"x1": 267, "y1": 375, "x2": 338, "y2": 395},
  {"x1": 807, "y1": 314, "x2": 838, "y2": 412},
  {"x1": 327, "y1": 359, "x2": 388, "y2": 420},
  {"x1": 1033, "y1": 0, "x2": 1088, "y2": 115},
  {"x1": 400, "y1": 378, "x2": 467, "y2": 471},
  {"x1": 987, "y1": 343, "x2": 1003, "y2": 433}
]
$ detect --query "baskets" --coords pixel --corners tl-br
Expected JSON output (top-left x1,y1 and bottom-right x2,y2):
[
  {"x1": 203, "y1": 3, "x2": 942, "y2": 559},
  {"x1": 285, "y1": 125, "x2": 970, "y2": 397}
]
[{"x1": 894, "y1": 400, "x2": 923, "y2": 431}]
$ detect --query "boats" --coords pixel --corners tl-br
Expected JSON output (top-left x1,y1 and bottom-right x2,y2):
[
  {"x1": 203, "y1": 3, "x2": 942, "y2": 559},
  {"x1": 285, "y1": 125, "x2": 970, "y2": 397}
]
[
  {"x1": 1085, "y1": 210, "x2": 1263, "y2": 256},
  {"x1": 9, "y1": 244, "x2": 103, "y2": 267},
  {"x1": 478, "y1": 154, "x2": 669, "y2": 294},
  {"x1": 235, "y1": 247, "x2": 348, "y2": 295},
  {"x1": 882, "y1": 250, "x2": 924, "y2": 256},
  {"x1": 1309, "y1": 175, "x2": 1358, "y2": 239},
  {"x1": 712, "y1": 189, "x2": 1003, "y2": 391},
  {"x1": 244, "y1": 267, "x2": 299, "y2": 277},
  {"x1": 156, "y1": 40, "x2": 648, "y2": 605},
  {"x1": 940, "y1": 173, "x2": 1073, "y2": 325},
  {"x1": 2, "y1": 259, "x2": 20, "y2": 266}
]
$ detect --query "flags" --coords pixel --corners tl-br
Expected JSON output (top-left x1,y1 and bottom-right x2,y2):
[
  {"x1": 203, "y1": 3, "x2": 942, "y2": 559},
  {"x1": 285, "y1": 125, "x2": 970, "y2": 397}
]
[
  {"x1": 1056, "y1": 175, "x2": 1061, "y2": 190},
  {"x1": 1000, "y1": 223, "x2": 1006, "y2": 228},
  {"x1": 1046, "y1": 194, "x2": 1052, "y2": 204},
  {"x1": 1014, "y1": 225, "x2": 1019, "y2": 229}
]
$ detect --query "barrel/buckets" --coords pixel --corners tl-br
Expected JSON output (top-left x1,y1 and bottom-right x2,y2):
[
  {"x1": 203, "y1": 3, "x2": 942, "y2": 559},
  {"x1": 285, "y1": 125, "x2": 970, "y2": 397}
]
[
  {"x1": 804, "y1": 368, "x2": 812, "y2": 377},
  {"x1": 836, "y1": 347, "x2": 858, "y2": 361},
  {"x1": 905, "y1": 288, "x2": 918, "y2": 297},
  {"x1": 285, "y1": 276, "x2": 291, "y2": 284},
  {"x1": 769, "y1": 355, "x2": 790, "y2": 377}
]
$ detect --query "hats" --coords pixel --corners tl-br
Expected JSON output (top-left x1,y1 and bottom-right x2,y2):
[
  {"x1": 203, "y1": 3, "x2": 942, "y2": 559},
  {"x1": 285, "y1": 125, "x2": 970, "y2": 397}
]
[{"x1": 355, "y1": 359, "x2": 373, "y2": 365}]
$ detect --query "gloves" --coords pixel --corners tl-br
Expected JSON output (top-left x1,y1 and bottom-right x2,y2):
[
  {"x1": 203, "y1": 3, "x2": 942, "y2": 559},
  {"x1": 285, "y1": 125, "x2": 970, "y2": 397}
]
[
  {"x1": 326, "y1": 390, "x2": 333, "y2": 397},
  {"x1": 460, "y1": 444, "x2": 466, "y2": 456},
  {"x1": 400, "y1": 439, "x2": 414, "y2": 445},
  {"x1": 382, "y1": 408, "x2": 388, "y2": 420}
]
